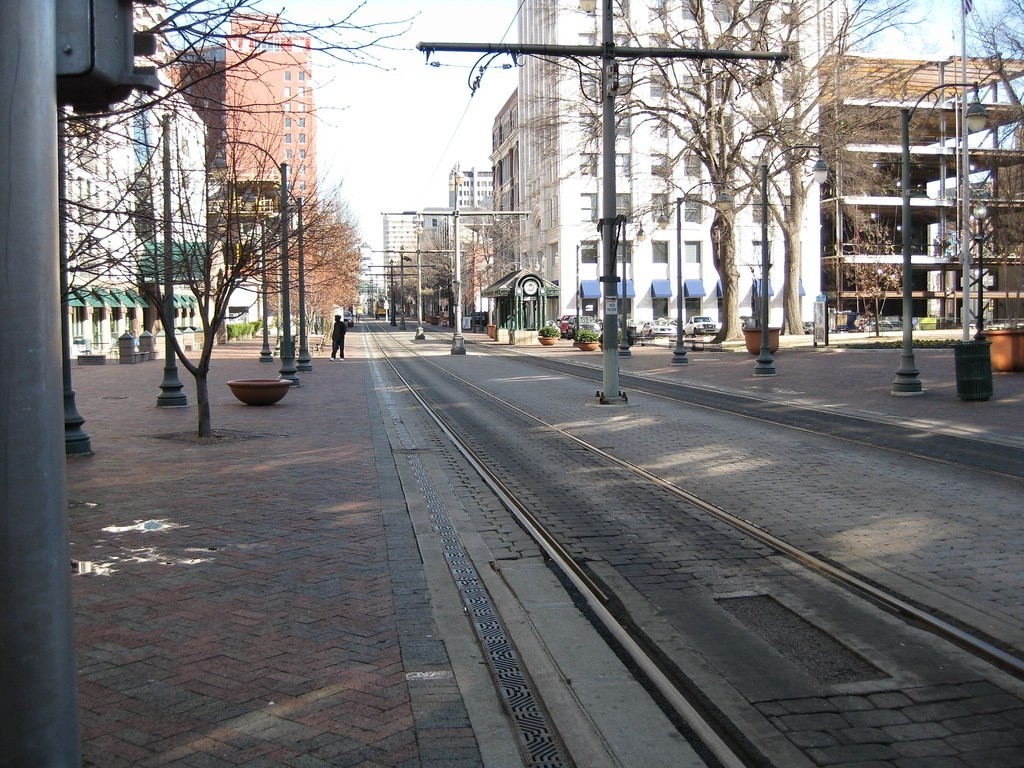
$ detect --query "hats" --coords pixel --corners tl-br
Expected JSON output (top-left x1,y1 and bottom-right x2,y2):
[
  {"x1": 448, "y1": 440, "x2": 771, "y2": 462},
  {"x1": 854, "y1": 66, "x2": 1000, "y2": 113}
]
[{"x1": 335, "y1": 315, "x2": 341, "y2": 319}]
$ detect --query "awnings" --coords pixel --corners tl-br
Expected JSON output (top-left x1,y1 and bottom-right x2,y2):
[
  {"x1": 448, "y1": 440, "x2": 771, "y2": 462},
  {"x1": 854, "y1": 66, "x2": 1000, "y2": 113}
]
[
  {"x1": 716, "y1": 280, "x2": 724, "y2": 298},
  {"x1": 190, "y1": 296, "x2": 196, "y2": 301},
  {"x1": 109, "y1": 288, "x2": 136, "y2": 307},
  {"x1": 92, "y1": 286, "x2": 121, "y2": 307},
  {"x1": 68, "y1": 293, "x2": 84, "y2": 306},
  {"x1": 756, "y1": 279, "x2": 774, "y2": 297},
  {"x1": 684, "y1": 279, "x2": 707, "y2": 298},
  {"x1": 799, "y1": 280, "x2": 806, "y2": 296},
  {"x1": 580, "y1": 280, "x2": 602, "y2": 299},
  {"x1": 126, "y1": 290, "x2": 149, "y2": 308},
  {"x1": 617, "y1": 279, "x2": 635, "y2": 299},
  {"x1": 173, "y1": 300, "x2": 178, "y2": 309},
  {"x1": 174, "y1": 294, "x2": 188, "y2": 309},
  {"x1": 652, "y1": 279, "x2": 673, "y2": 297},
  {"x1": 182, "y1": 295, "x2": 196, "y2": 310},
  {"x1": 76, "y1": 285, "x2": 104, "y2": 307}
]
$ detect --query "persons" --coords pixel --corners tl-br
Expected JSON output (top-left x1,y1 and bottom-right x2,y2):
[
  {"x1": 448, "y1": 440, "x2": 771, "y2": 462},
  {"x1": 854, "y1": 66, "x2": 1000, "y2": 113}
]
[{"x1": 330, "y1": 315, "x2": 347, "y2": 361}]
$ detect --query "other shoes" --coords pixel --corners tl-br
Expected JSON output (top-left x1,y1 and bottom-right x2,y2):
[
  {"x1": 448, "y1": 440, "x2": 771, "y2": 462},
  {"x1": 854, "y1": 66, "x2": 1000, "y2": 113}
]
[
  {"x1": 339, "y1": 358, "x2": 345, "y2": 362},
  {"x1": 330, "y1": 358, "x2": 336, "y2": 361}
]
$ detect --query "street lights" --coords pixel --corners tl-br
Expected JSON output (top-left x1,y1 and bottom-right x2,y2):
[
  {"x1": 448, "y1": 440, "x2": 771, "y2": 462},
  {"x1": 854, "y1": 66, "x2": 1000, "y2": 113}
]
[
  {"x1": 414, "y1": 221, "x2": 425, "y2": 340},
  {"x1": 619, "y1": 205, "x2": 669, "y2": 360},
  {"x1": 891, "y1": 81, "x2": 990, "y2": 397},
  {"x1": 210, "y1": 141, "x2": 301, "y2": 389},
  {"x1": 625, "y1": 221, "x2": 646, "y2": 241},
  {"x1": 448, "y1": 160, "x2": 467, "y2": 355},
  {"x1": 669, "y1": 178, "x2": 733, "y2": 365},
  {"x1": 752, "y1": 145, "x2": 829, "y2": 378}
]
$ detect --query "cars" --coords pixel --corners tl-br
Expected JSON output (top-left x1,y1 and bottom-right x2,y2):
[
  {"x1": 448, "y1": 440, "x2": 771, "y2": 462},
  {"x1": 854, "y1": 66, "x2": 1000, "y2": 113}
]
[
  {"x1": 641, "y1": 320, "x2": 677, "y2": 338},
  {"x1": 566, "y1": 315, "x2": 601, "y2": 340},
  {"x1": 683, "y1": 316, "x2": 722, "y2": 338},
  {"x1": 556, "y1": 314, "x2": 577, "y2": 338}
]
